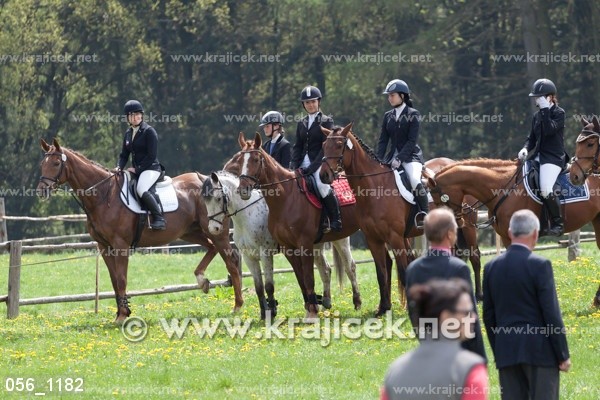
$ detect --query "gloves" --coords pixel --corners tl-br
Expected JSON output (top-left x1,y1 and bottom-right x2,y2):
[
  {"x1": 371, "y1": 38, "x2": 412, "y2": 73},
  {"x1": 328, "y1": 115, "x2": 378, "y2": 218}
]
[
  {"x1": 518, "y1": 148, "x2": 528, "y2": 159},
  {"x1": 297, "y1": 167, "x2": 311, "y2": 176},
  {"x1": 536, "y1": 96, "x2": 550, "y2": 109},
  {"x1": 389, "y1": 158, "x2": 401, "y2": 171},
  {"x1": 288, "y1": 161, "x2": 298, "y2": 172}
]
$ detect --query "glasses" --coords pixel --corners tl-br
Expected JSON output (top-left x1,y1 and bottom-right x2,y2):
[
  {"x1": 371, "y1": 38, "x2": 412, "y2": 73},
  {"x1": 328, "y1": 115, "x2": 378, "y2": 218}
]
[{"x1": 456, "y1": 309, "x2": 473, "y2": 317}]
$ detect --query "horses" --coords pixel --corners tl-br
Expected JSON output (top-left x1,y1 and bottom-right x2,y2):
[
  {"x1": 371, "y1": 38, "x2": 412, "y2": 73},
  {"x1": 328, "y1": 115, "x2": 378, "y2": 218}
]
[
  {"x1": 196, "y1": 170, "x2": 362, "y2": 326},
  {"x1": 421, "y1": 156, "x2": 600, "y2": 311},
  {"x1": 35, "y1": 137, "x2": 245, "y2": 325},
  {"x1": 318, "y1": 120, "x2": 485, "y2": 318},
  {"x1": 238, "y1": 131, "x2": 418, "y2": 326},
  {"x1": 569, "y1": 112, "x2": 600, "y2": 187}
]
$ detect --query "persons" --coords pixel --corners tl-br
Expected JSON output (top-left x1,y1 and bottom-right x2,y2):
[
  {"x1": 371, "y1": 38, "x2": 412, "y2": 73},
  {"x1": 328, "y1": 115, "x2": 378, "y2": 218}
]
[
  {"x1": 404, "y1": 206, "x2": 488, "y2": 364},
  {"x1": 374, "y1": 80, "x2": 431, "y2": 229},
  {"x1": 260, "y1": 111, "x2": 291, "y2": 171},
  {"x1": 482, "y1": 209, "x2": 571, "y2": 400},
  {"x1": 287, "y1": 84, "x2": 344, "y2": 237},
  {"x1": 114, "y1": 100, "x2": 167, "y2": 230},
  {"x1": 380, "y1": 275, "x2": 490, "y2": 400},
  {"x1": 518, "y1": 79, "x2": 567, "y2": 235}
]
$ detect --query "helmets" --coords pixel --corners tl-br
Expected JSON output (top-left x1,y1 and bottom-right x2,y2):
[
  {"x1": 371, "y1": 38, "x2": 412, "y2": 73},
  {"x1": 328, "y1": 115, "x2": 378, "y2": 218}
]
[
  {"x1": 123, "y1": 100, "x2": 143, "y2": 115},
  {"x1": 528, "y1": 78, "x2": 557, "y2": 97},
  {"x1": 298, "y1": 85, "x2": 323, "y2": 101},
  {"x1": 382, "y1": 78, "x2": 409, "y2": 94},
  {"x1": 258, "y1": 110, "x2": 285, "y2": 127}
]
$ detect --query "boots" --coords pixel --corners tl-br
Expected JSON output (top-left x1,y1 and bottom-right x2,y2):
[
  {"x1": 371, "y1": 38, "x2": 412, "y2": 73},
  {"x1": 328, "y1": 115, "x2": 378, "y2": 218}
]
[
  {"x1": 321, "y1": 188, "x2": 343, "y2": 233},
  {"x1": 541, "y1": 192, "x2": 564, "y2": 240},
  {"x1": 140, "y1": 191, "x2": 167, "y2": 230},
  {"x1": 412, "y1": 184, "x2": 430, "y2": 225}
]
